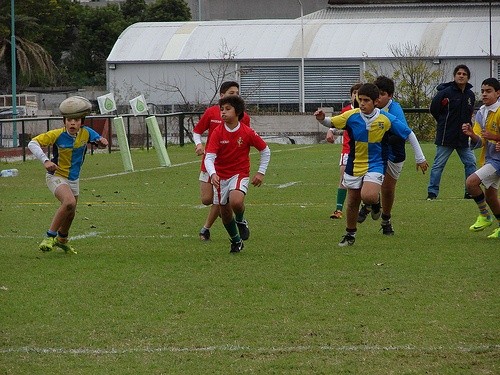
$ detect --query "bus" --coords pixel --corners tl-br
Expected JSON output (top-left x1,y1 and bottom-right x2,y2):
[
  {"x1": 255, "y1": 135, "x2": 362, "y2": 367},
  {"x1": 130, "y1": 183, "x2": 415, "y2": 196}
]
[{"x1": 0, "y1": 94, "x2": 39, "y2": 120}]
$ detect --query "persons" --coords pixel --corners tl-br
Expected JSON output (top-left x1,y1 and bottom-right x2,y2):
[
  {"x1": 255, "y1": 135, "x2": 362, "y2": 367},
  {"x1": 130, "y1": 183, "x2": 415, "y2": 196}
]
[
  {"x1": 462, "y1": 78, "x2": 500, "y2": 239},
  {"x1": 313, "y1": 83, "x2": 430, "y2": 247},
  {"x1": 325, "y1": 83, "x2": 363, "y2": 219},
  {"x1": 495, "y1": 141, "x2": 500, "y2": 152},
  {"x1": 356, "y1": 75, "x2": 409, "y2": 236},
  {"x1": 204, "y1": 95, "x2": 271, "y2": 253},
  {"x1": 425, "y1": 64, "x2": 476, "y2": 201},
  {"x1": 27, "y1": 116, "x2": 109, "y2": 254},
  {"x1": 191, "y1": 81, "x2": 251, "y2": 241}
]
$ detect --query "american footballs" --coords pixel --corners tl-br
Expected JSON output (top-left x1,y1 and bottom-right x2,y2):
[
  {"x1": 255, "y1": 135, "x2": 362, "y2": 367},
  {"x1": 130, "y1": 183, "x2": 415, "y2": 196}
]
[{"x1": 57, "y1": 96, "x2": 93, "y2": 119}]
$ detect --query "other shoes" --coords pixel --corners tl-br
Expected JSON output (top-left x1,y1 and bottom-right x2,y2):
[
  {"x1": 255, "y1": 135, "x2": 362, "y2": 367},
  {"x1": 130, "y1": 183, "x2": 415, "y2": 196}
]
[
  {"x1": 426, "y1": 192, "x2": 437, "y2": 201},
  {"x1": 464, "y1": 190, "x2": 474, "y2": 199}
]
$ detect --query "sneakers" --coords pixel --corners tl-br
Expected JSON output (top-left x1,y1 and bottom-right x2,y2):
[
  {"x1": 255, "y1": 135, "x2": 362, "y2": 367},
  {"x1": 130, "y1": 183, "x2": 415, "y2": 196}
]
[
  {"x1": 378, "y1": 216, "x2": 395, "y2": 236},
  {"x1": 487, "y1": 226, "x2": 500, "y2": 239},
  {"x1": 357, "y1": 202, "x2": 371, "y2": 224},
  {"x1": 39, "y1": 236, "x2": 54, "y2": 253},
  {"x1": 338, "y1": 230, "x2": 357, "y2": 247},
  {"x1": 54, "y1": 237, "x2": 78, "y2": 255},
  {"x1": 235, "y1": 218, "x2": 250, "y2": 240},
  {"x1": 227, "y1": 237, "x2": 244, "y2": 254},
  {"x1": 469, "y1": 213, "x2": 493, "y2": 232},
  {"x1": 369, "y1": 193, "x2": 382, "y2": 221},
  {"x1": 199, "y1": 229, "x2": 210, "y2": 241},
  {"x1": 330, "y1": 209, "x2": 343, "y2": 220}
]
